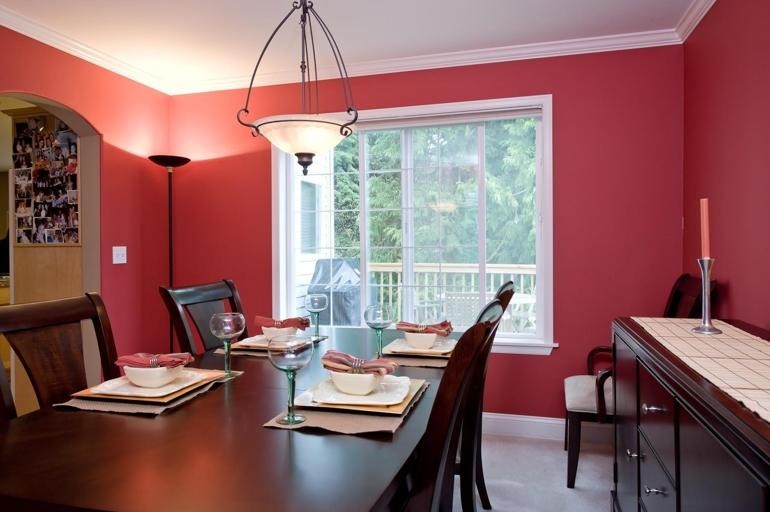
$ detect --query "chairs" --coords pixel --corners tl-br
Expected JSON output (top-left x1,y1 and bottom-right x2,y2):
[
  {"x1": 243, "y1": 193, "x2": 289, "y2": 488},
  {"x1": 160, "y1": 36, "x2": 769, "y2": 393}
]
[
  {"x1": 563, "y1": 272, "x2": 717, "y2": 489},
  {"x1": 457, "y1": 282, "x2": 514, "y2": 511},
  {"x1": 0, "y1": 292, "x2": 121, "y2": 423},
  {"x1": 390, "y1": 299, "x2": 503, "y2": 512},
  {"x1": 158, "y1": 280, "x2": 249, "y2": 356}
]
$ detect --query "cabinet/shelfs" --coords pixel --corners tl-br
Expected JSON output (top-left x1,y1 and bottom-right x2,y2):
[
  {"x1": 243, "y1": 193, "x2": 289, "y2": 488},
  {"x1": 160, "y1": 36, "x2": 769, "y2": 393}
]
[{"x1": 610, "y1": 317, "x2": 770, "y2": 512}]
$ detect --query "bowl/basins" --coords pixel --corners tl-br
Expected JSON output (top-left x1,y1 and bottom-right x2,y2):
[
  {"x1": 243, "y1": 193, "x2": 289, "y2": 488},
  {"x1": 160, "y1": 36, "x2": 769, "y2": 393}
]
[
  {"x1": 329, "y1": 370, "x2": 385, "y2": 396},
  {"x1": 403, "y1": 331, "x2": 438, "y2": 348},
  {"x1": 123, "y1": 365, "x2": 184, "y2": 388}
]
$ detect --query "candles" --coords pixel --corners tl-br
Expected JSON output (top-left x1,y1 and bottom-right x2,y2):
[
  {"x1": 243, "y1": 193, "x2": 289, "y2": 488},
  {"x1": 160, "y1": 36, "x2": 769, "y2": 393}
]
[{"x1": 700, "y1": 198, "x2": 711, "y2": 258}]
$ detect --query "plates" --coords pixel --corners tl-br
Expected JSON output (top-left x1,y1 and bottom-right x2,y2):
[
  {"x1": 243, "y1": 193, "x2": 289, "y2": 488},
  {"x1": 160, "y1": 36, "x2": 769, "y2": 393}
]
[
  {"x1": 383, "y1": 337, "x2": 459, "y2": 355},
  {"x1": 91, "y1": 370, "x2": 208, "y2": 398},
  {"x1": 317, "y1": 376, "x2": 409, "y2": 407},
  {"x1": 239, "y1": 333, "x2": 315, "y2": 349},
  {"x1": 261, "y1": 325, "x2": 299, "y2": 343}
]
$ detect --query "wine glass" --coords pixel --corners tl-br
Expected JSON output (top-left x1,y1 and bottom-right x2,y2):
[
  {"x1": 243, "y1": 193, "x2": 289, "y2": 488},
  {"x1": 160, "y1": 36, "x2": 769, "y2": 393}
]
[
  {"x1": 304, "y1": 293, "x2": 328, "y2": 342},
  {"x1": 268, "y1": 336, "x2": 314, "y2": 425},
  {"x1": 364, "y1": 305, "x2": 394, "y2": 361},
  {"x1": 209, "y1": 314, "x2": 245, "y2": 379}
]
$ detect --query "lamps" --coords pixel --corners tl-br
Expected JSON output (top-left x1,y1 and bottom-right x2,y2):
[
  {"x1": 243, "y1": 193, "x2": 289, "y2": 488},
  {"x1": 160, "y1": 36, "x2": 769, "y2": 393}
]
[
  {"x1": 236, "y1": 0, "x2": 358, "y2": 175},
  {"x1": 147, "y1": 155, "x2": 190, "y2": 353}
]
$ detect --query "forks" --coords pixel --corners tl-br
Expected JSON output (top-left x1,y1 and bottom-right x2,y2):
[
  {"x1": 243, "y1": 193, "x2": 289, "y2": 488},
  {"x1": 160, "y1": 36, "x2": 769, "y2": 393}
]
[
  {"x1": 148, "y1": 355, "x2": 160, "y2": 369},
  {"x1": 418, "y1": 322, "x2": 427, "y2": 334},
  {"x1": 274, "y1": 319, "x2": 283, "y2": 329},
  {"x1": 350, "y1": 357, "x2": 365, "y2": 375}
]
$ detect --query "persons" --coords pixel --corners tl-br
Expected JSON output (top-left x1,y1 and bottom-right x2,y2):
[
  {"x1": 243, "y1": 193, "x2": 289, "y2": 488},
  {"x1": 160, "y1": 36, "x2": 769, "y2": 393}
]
[{"x1": 12, "y1": 115, "x2": 78, "y2": 243}]
